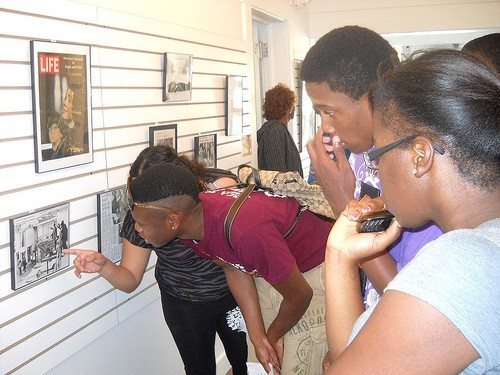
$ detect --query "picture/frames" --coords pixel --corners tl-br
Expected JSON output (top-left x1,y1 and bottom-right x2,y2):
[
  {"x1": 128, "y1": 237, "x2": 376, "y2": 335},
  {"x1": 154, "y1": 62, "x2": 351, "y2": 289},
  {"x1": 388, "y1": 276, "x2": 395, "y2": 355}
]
[
  {"x1": 193, "y1": 134, "x2": 217, "y2": 169},
  {"x1": 149, "y1": 124, "x2": 177, "y2": 153},
  {"x1": 29, "y1": 39, "x2": 94, "y2": 174}
]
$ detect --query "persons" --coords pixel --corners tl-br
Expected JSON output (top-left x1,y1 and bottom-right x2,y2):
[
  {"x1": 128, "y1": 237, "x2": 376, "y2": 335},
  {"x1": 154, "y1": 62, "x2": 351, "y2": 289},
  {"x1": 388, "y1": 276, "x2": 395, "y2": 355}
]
[
  {"x1": 322, "y1": 33, "x2": 500, "y2": 375},
  {"x1": 301, "y1": 25, "x2": 444, "y2": 312},
  {"x1": 256, "y1": 85, "x2": 304, "y2": 179},
  {"x1": 47, "y1": 85, "x2": 89, "y2": 160},
  {"x1": 62, "y1": 145, "x2": 248, "y2": 375},
  {"x1": 461, "y1": 33, "x2": 500, "y2": 80},
  {"x1": 126, "y1": 162, "x2": 337, "y2": 375}
]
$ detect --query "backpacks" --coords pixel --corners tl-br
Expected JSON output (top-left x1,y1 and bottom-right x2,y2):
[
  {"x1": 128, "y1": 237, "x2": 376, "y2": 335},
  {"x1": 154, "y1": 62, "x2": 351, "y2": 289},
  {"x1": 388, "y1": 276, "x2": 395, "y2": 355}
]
[{"x1": 222, "y1": 164, "x2": 337, "y2": 252}]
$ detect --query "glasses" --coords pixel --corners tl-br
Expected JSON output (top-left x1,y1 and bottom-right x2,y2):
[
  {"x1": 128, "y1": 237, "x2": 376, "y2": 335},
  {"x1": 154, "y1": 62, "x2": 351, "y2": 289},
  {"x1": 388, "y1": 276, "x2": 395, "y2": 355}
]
[
  {"x1": 126, "y1": 177, "x2": 180, "y2": 215},
  {"x1": 362, "y1": 134, "x2": 446, "y2": 170}
]
